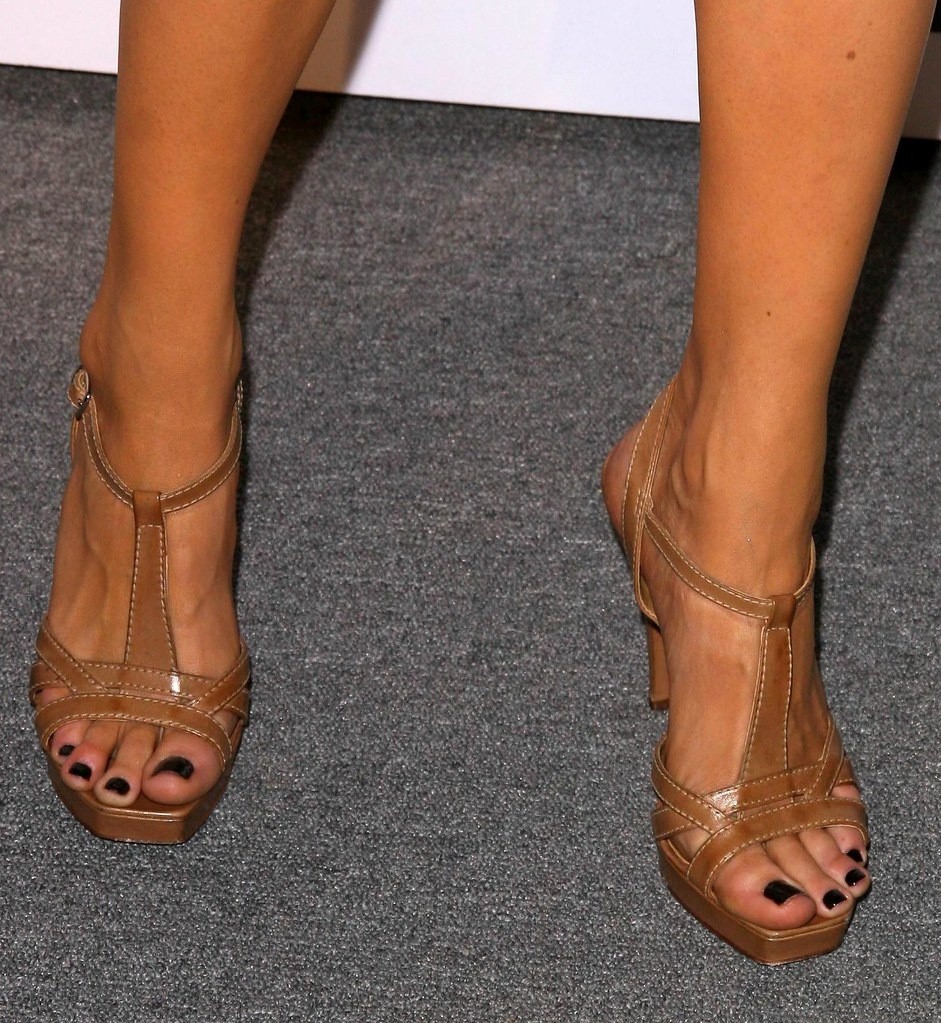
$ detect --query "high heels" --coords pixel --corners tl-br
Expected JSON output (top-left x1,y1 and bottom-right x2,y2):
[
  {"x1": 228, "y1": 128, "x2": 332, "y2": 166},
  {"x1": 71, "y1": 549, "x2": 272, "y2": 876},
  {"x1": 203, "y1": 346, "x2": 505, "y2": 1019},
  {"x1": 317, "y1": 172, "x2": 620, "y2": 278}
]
[
  {"x1": 603, "y1": 379, "x2": 868, "y2": 965},
  {"x1": 28, "y1": 344, "x2": 249, "y2": 844}
]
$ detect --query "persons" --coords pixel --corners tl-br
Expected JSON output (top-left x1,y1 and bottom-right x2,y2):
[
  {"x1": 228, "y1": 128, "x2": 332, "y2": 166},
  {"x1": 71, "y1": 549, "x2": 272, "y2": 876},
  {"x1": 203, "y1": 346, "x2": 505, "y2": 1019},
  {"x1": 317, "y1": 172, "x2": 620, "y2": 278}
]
[{"x1": 29, "y1": 0, "x2": 937, "y2": 966}]
